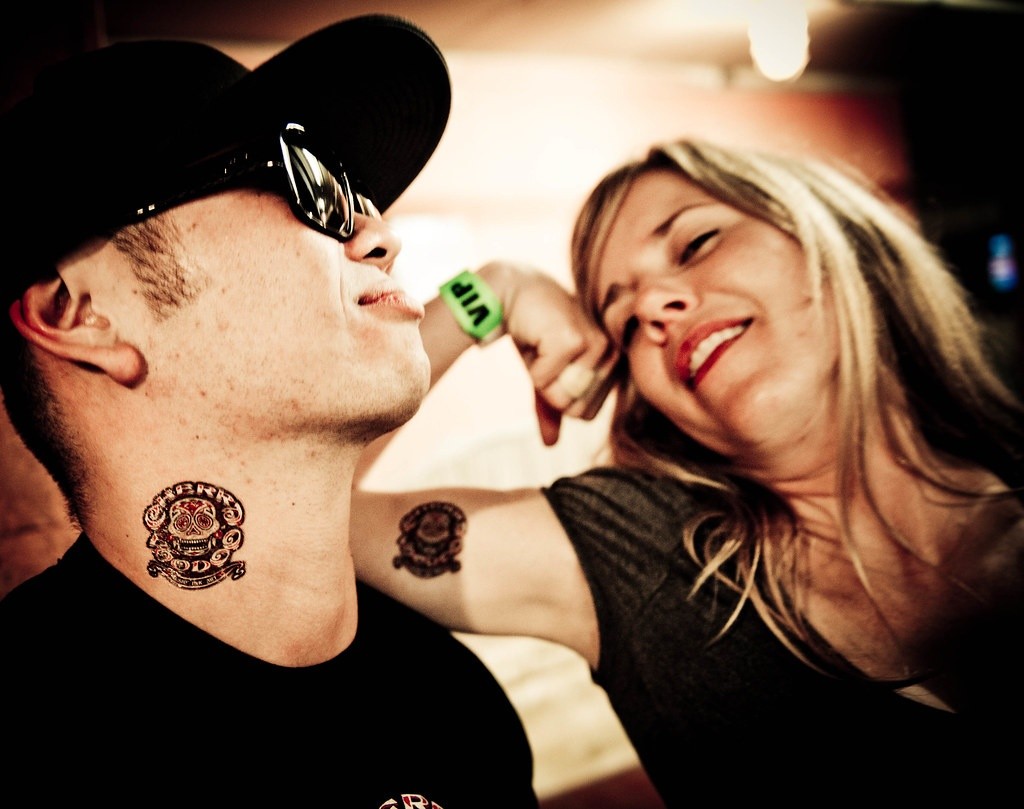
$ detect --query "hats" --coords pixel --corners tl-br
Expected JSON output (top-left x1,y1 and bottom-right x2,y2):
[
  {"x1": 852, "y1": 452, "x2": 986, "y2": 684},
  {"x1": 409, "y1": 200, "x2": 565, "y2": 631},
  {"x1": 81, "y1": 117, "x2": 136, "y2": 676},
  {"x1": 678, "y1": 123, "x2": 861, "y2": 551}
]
[{"x1": 1, "y1": 12, "x2": 453, "y2": 311}]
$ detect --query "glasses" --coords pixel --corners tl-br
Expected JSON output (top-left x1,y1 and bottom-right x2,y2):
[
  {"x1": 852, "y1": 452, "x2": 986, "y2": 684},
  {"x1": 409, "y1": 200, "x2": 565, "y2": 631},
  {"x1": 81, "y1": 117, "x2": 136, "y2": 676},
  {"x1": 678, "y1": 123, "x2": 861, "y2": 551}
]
[{"x1": 131, "y1": 122, "x2": 383, "y2": 244}]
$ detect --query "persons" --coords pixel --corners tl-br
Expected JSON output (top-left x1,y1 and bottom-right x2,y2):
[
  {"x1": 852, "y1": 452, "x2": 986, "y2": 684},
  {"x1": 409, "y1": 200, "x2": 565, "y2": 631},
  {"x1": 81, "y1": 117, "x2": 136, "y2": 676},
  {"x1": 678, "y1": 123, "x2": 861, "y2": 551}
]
[
  {"x1": 1, "y1": 11, "x2": 539, "y2": 809},
  {"x1": 348, "y1": 129, "x2": 1024, "y2": 809}
]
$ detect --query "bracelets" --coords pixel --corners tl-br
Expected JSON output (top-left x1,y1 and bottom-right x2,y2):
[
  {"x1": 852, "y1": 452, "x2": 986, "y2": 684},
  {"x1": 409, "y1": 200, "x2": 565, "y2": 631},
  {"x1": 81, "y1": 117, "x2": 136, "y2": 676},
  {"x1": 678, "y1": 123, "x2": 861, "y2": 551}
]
[{"x1": 440, "y1": 269, "x2": 506, "y2": 346}]
